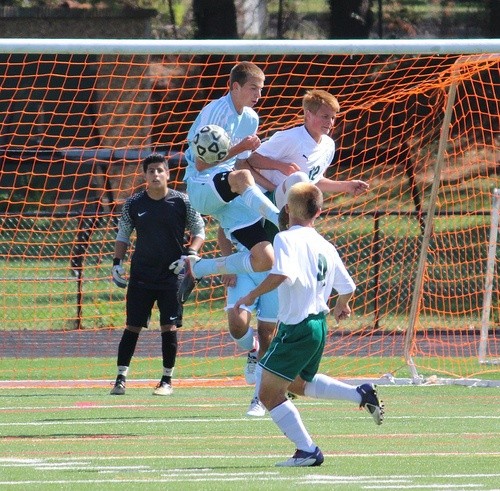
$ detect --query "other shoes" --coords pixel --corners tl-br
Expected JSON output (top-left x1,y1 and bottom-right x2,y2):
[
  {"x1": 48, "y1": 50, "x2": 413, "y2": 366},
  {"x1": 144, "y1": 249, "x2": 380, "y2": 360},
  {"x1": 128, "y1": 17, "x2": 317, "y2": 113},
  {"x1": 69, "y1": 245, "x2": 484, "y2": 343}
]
[
  {"x1": 109, "y1": 378, "x2": 126, "y2": 397},
  {"x1": 152, "y1": 377, "x2": 176, "y2": 398}
]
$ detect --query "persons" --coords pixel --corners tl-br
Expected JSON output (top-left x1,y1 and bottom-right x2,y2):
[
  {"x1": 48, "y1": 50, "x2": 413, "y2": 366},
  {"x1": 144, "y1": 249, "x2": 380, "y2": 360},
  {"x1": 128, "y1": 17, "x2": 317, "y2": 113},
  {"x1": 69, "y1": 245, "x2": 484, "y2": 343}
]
[
  {"x1": 176, "y1": 61, "x2": 370, "y2": 418},
  {"x1": 233, "y1": 181, "x2": 385, "y2": 467},
  {"x1": 109, "y1": 153, "x2": 205, "y2": 396}
]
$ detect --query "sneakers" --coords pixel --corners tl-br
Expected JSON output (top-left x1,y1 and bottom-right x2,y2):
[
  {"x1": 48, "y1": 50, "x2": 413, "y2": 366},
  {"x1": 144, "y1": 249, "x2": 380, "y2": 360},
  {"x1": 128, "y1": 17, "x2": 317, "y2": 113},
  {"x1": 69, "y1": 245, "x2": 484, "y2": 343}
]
[
  {"x1": 245, "y1": 355, "x2": 258, "y2": 385},
  {"x1": 270, "y1": 446, "x2": 324, "y2": 467},
  {"x1": 245, "y1": 396, "x2": 266, "y2": 419},
  {"x1": 356, "y1": 382, "x2": 383, "y2": 428},
  {"x1": 175, "y1": 254, "x2": 202, "y2": 305}
]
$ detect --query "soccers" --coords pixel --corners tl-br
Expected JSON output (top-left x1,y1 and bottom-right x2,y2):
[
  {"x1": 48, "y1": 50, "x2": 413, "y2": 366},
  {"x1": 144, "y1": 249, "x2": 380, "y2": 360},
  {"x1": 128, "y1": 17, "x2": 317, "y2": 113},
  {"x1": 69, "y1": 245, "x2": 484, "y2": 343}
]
[{"x1": 192, "y1": 124, "x2": 232, "y2": 165}]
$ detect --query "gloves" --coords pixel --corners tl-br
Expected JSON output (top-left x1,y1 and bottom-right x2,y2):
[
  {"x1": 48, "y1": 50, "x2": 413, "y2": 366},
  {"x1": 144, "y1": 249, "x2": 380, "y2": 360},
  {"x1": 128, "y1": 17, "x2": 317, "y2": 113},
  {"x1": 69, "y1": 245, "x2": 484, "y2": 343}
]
[
  {"x1": 168, "y1": 248, "x2": 198, "y2": 278},
  {"x1": 111, "y1": 258, "x2": 128, "y2": 288}
]
